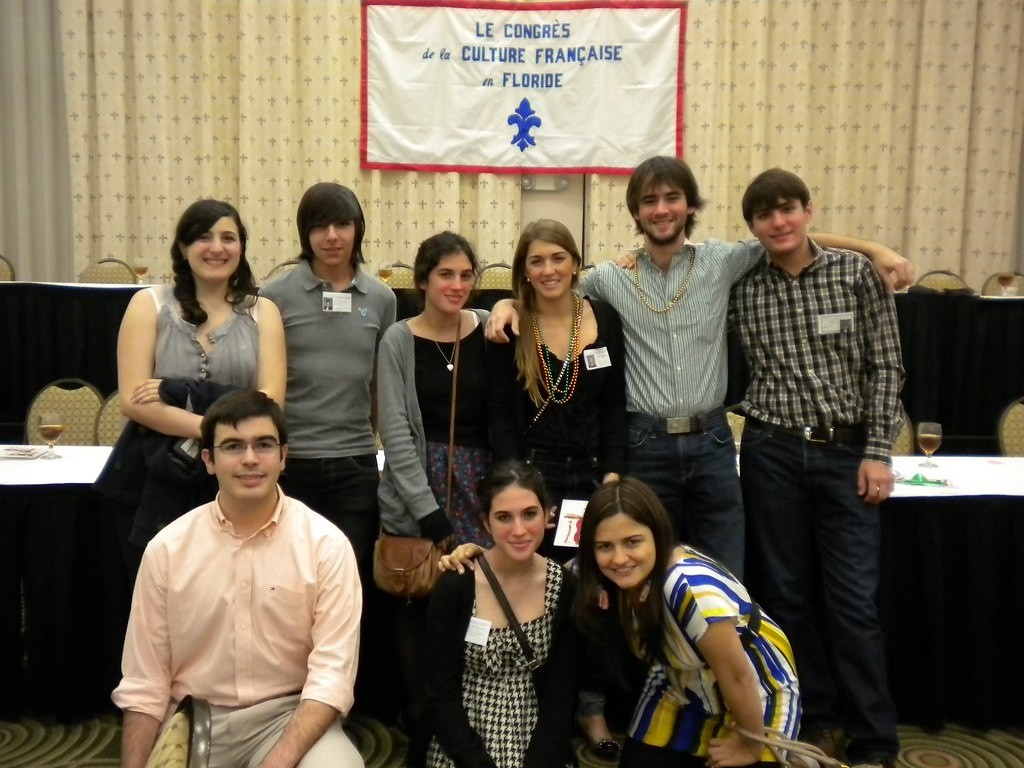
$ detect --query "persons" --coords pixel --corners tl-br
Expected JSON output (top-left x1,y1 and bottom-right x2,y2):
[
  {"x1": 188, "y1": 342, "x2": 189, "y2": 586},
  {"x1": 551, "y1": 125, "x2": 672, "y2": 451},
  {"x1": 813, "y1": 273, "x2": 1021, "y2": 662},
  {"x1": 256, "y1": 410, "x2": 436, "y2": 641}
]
[
  {"x1": 111, "y1": 389, "x2": 365, "y2": 768},
  {"x1": 727, "y1": 168, "x2": 906, "y2": 768},
  {"x1": 376, "y1": 231, "x2": 490, "y2": 739},
  {"x1": 484, "y1": 157, "x2": 915, "y2": 586},
  {"x1": 93, "y1": 200, "x2": 287, "y2": 598},
  {"x1": 259, "y1": 183, "x2": 397, "y2": 715},
  {"x1": 407, "y1": 457, "x2": 803, "y2": 768},
  {"x1": 488, "y1": 225, "x2": 626, "y2": 761}
]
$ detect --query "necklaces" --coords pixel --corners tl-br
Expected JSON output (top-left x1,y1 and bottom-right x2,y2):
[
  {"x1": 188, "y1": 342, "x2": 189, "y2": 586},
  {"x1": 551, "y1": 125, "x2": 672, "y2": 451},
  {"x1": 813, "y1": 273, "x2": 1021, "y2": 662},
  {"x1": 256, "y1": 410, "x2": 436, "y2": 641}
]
[
  {"x1": 421, "y1": 311, "x2": 456, "y2": 371},
  {"x1": 531, "y1": 291, "x2": 584, "y2": 405},
  {"x1": 633, "y1": 247, "x2": 695, "y2": 313}
]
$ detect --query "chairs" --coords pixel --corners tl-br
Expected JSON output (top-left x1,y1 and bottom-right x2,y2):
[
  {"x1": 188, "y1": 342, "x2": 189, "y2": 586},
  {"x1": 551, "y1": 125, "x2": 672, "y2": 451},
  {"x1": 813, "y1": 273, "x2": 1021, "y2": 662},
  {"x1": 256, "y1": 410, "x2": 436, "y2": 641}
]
[{"x1": 1, "y1": 255, "x2": 1024, "y2": 457}]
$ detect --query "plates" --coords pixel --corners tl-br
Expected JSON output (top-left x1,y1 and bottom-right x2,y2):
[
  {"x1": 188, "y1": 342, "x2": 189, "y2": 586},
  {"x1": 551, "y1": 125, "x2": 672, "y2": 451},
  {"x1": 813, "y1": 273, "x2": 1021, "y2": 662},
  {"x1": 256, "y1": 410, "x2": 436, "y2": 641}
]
[
  {"x1": 0, "y1": 445, "x2": 47, "y2": 459},
  {"x1": 892, "y1": 469, "x2": 904, "y2": 481}
]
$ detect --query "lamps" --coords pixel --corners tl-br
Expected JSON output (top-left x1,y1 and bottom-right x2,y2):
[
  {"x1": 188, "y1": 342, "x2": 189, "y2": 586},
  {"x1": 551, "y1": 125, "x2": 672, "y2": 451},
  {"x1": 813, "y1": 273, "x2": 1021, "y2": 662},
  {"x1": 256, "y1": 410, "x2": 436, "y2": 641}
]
[{"x1": 520, "y1": 174, "x2": 569, "y2": 192}]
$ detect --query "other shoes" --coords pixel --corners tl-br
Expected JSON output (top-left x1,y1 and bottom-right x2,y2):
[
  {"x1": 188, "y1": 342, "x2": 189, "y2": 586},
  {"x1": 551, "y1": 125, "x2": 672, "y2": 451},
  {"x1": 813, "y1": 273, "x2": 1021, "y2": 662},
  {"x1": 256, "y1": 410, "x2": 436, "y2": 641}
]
[
  {"x1": 575, "y1": 715, "x2": 619, "y2": 758},
  {"x1": 800, "y1": 727, "x2": 837, "y2": 759},
  {"x1": 854, "y1": 759, "x2": 888, "y2": 768}
]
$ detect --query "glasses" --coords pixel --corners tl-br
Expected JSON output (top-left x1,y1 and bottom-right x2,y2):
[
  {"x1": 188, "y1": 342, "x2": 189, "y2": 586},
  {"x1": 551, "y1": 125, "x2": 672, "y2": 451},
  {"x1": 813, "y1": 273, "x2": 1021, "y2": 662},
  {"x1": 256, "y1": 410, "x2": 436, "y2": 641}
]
[{"x1": 209, "y1": 438, "x2": 282, "y2": 455}]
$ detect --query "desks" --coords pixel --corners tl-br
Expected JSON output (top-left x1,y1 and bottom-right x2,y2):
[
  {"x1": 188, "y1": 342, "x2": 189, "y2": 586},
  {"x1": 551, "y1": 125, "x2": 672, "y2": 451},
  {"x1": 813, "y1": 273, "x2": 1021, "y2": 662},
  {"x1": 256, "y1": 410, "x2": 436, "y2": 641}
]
[
  {"x1": 0, "y1": 278, "x2": 158, "y2": 448},
  {"x1": 732, "y1": 454, "x2": 1024, "y2": 741},
  {"x1": 894, "y1": 292, "x2": 1024, "y2": 452},
  {"x1": 0, "y1": 444, "x2": 150, "y2": 724},
  {"x1": 393, "y1": 284, "x2": 516, "y2": 321}
]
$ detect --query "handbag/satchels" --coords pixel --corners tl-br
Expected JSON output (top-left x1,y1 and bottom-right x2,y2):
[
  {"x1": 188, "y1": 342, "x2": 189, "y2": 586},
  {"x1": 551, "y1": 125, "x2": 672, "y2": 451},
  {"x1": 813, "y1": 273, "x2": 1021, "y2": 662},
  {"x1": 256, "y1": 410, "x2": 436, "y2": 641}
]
[
  {"x1": 735, "y1": 725, "x2": 848, "y2": 768},
  {"x1": 531, "y1": 655, "x2": 580, "y2": 721},
  {"x1": 373, "y1": 534, "x2": 444, "y2": 601}
]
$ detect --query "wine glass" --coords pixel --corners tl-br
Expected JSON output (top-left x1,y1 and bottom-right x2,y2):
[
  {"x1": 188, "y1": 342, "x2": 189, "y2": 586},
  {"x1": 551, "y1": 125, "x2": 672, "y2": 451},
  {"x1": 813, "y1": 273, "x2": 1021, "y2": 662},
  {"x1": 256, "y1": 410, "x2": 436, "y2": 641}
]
[
  {"x1": 38, "y1": 413, "x2": 63, "y2": 460},
  {"x1": 917, "y1": 422, "x2": 941, "y2": 468}
]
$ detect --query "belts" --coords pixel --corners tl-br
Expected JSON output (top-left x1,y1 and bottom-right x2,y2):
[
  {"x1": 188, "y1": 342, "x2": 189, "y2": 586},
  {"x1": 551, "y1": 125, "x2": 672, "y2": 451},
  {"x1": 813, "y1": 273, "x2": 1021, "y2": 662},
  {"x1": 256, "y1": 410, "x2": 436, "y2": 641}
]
[
  {"x1": 627, "y1": 406, "x2": 728, "y2": 433},
  {"x1": 745, "y1": 413, "x2": 866, "y2": 443}
]
